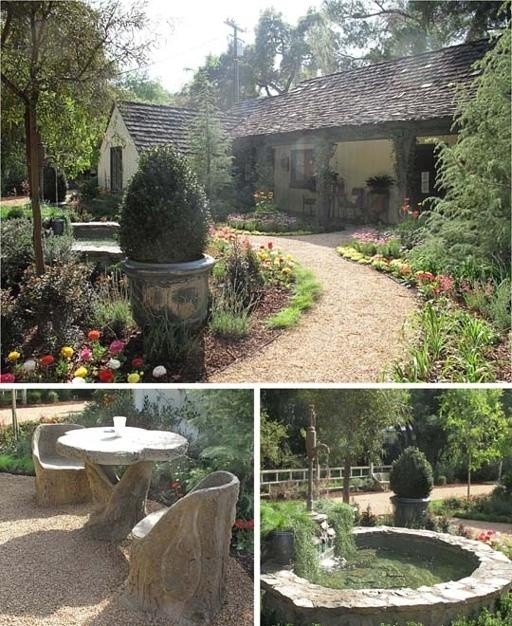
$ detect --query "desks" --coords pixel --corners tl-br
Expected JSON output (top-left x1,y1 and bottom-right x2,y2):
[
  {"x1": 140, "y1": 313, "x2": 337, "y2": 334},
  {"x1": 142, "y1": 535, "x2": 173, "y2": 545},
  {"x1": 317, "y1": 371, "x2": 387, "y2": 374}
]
[{"x1": 56, "y1": 426, "x2": 188, "y2": 542}]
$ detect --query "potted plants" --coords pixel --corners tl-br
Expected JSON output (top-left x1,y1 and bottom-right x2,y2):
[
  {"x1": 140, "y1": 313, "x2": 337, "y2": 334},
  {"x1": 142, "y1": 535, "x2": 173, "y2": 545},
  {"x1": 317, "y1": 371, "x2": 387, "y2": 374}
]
[
  {"x1": 260, "y1": 502, "x2": 316, "y2": 566},
  {"x1": 390, "y1": 446, "x2": 435, "y2": 528},
  {"x1": 366, "y1": 173, "x2": 397, "y2": 209},
  {"x1": 116, "y1": 147, "x2": 218, "y2": 336}
]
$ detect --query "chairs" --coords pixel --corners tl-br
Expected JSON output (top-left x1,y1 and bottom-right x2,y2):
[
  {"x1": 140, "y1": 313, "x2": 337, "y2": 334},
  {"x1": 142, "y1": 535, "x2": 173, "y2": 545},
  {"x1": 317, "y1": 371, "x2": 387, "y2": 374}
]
[
  {"x1": 31, "y1": 423, "x2": 92, "y2": 506},
  {"x1": 129, "y1": 472, "x2": 241, "y2": 620}
]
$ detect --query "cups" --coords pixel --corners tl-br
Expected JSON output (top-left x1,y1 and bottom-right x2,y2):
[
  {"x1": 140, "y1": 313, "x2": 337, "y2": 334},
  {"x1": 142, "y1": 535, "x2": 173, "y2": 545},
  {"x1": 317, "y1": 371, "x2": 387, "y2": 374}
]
[{"x1": 112, "y1": 416, "x2": 126, "y2": 439}]
